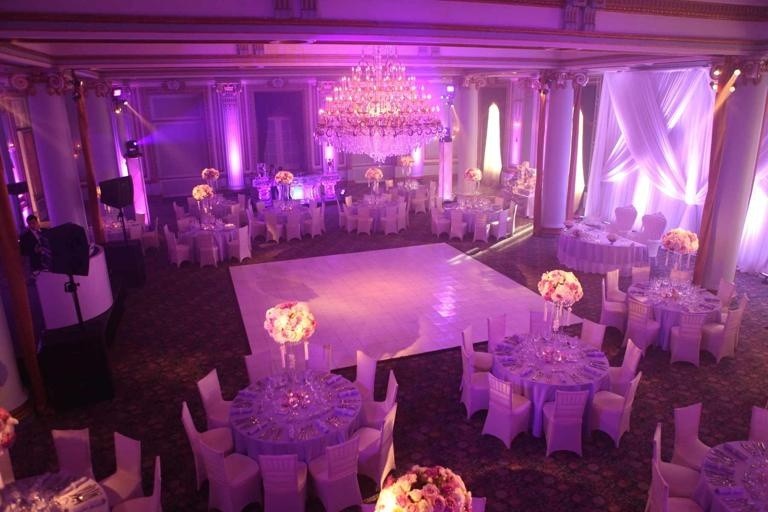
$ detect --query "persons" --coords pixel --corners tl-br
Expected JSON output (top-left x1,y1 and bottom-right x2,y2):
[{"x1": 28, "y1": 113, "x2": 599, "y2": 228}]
[{"x1": 18, "y1": 215, "x2": 48, "y2": 270}]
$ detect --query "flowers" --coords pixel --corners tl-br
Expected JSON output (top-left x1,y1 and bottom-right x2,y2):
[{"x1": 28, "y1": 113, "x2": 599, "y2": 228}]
[
  {"x1": 275, "y1": 170, "x2": 293, "y2": 184},
  {"x1": 398, "y1": 156, "x2": 415, "y2": 167},
  {"x1": 464, "y1": 167, "x2": 482, "y2": 181},
  {"x1": 201, "y1": 168, "x2": 219, "y2": 180},
  {"x1": 382, "y1": 463, "x2": 473, "y2": 512},
  {"x1": 0, "y1": 406, "x2": 19, "y2": 449},
  {"x1": 365, "y1": 167, "x2": 383, "y2": 183}
]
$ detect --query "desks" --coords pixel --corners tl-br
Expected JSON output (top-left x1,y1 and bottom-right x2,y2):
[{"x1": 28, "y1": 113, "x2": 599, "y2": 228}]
[
  {"x1": 498, "y1": 333, "x2": 610, "y2": 439},
  {"x1": 231, "y1": 367, "x2": 363, "y2": 457},
  {"x1": 699, "y1": 439, "x2": 766, "y2": 510}
]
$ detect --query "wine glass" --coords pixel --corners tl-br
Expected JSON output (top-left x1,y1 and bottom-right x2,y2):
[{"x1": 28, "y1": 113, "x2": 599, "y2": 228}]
[
  {"x1": 518, "y1": 326, "x2": 580, "y2": 367},
  {"x1": 265, "y1": 370, "x2": 334, "y2": 422},
  {"x1": 651, "y1": 274, "x2": 697, "y2": 306}
]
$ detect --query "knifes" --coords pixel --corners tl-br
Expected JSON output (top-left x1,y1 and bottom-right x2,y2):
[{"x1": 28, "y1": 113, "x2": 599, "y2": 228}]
[
  {"x1": 67, "y1": 484, "x2": 101, "y2": 505},
  {"x1": 60, "y1": 474, "x2": 91, "y2": 495}
]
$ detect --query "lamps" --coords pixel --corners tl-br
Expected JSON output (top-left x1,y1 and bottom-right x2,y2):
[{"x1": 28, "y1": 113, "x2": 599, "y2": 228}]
[
  {"x1": 112, "y1": 87, "x2": 129, "y2": 114},
  {"x1": 709, "y1": 65, "x2": 742, "y2": 93},
  {"x1": 446, "y1": 85, "x2": 456, "y2": 94},
  {"x1": 312, "y1": 47, "x2": 445, "y2": 165}
]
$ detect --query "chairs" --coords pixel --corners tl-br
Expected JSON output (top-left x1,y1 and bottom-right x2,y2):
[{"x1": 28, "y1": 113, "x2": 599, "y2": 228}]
[
  {"x1": 529, "y1": 309, "x2": 556, "y2": 335},
  {"x1": 352, "y1": 403, "x2": 398, "y2": 485},
  {"x1": 461, "y1": 325, "x2": 493, "y2": 370},
  {"x1": 53, "y1": 429, "x2": 95, "y2": 480},
  {"x1": 272, "y1": 342, "x2": 306, "y2": 370},
  {"x1": 670, "y1": 402, "x2": 710, "y2": 469},
  {"x1": 590, "y1": 371, "x2": 644, "y2": 448},
  {"x1": 309, "y1": 434, "x2": 363, "y2": 510},
  {"x1": 557, "y1": 203, "x2": 750, "y2": 368},
  {"x1": 52, "y1": 179, "x2": 519, "y2": 267},
  {"x1": 243, "y1": 349, "x2": 273, "y2": 383},
  {"x1": 196, "y1": 368, "x2": 232, "y2": 428},
  {"x1": 480, "y1": 373, "x2": 531, "y2": 447},
  {"x1": 114, "y1": 456, "x2": 163, "y2": 511},
  {"x1": 750, "y1": 404, "x2": 768, "y2": 444},
  {"x1": 360, "y1": 369, "x2": 400, "y2": 425},
  {"x1": 352, "y1": 349, "x2": 379, "y2": 400},
  {"x1": 181, "y1": 400, "x2": 235, "y2": 491},
  {"x1": 646, "y1": 462, "x2": 701, "y2": 511},
  {"x1": 100, "y1": 431, "x2": 145, "y2": 500},
  {"x1": 651, "y1": 422, "x2": 699, "y2": 496},
  {"x1": 459, "y1": 345, "x2": 496, "y2": 419},
  {"x1": 256, "y1": 451, "x2": 309, "y2": 510},
  {"x1": 608, "y1": 338, "x2": 643, "y2": 392},
  {"x1": 485, "y1": 311, "x2": 506, "y2": 352},
  {"x1": 195, "y1": 438, "x2": 261, "y2": 509},
  {"x1": 304, "y1": 341, "x2": 333, "y2": 373},
  {"x1": 541, "y1": 389, "x2": 587, "y2": 456}
]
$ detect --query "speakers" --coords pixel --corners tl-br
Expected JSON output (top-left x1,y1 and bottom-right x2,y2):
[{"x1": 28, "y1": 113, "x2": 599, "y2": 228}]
[
  {"x1": 100, "y1": 176, "x2": 131, "y2": 208},
  {"x1": 40, "y1": 223, "x2": 89, "y2": 275}
]
